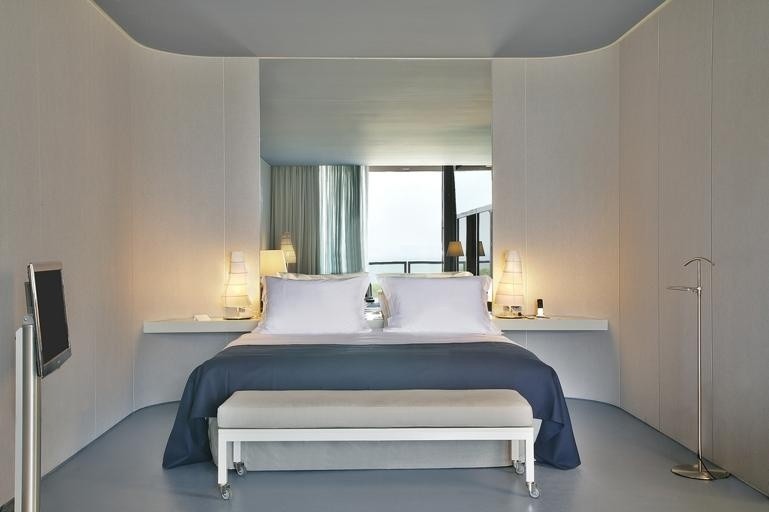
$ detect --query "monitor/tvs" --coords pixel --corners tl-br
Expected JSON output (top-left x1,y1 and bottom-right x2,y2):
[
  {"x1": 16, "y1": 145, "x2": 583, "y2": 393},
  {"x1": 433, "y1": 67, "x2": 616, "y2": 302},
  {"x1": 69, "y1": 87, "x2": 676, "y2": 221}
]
[{"x1": 27, "y1": 260, "x2": 72, "y2": 379}]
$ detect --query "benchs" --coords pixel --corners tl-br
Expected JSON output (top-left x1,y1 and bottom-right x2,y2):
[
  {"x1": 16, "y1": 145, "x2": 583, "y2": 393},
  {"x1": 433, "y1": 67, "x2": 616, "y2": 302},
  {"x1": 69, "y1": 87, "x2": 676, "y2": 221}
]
[{"x1": 216, "y1": 389, "x2": 540, "y2": 499}]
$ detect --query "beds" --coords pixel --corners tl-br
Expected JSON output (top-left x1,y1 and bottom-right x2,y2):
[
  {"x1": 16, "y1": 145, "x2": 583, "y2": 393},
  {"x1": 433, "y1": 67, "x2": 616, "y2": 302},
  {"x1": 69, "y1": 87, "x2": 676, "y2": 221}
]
[{"x1": 162, "y1": 273, "x2": 581, "y2": 470}]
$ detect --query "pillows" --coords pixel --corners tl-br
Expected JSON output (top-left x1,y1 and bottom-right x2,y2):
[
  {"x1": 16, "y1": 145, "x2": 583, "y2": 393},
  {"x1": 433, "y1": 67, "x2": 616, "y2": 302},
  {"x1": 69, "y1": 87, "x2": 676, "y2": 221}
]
[
  {"x1": 252, "y1": 275, "x2": 368, "y2": 335},
  {"x1": 375, "y1": 275, "x2": 503, "y2": 335}
]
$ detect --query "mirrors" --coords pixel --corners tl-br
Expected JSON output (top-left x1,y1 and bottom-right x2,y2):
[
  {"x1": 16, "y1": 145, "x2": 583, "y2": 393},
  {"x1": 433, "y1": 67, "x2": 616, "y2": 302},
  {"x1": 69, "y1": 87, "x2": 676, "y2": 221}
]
[{"x1": 260, "y1": 59, "x2": 493, "y2": 318}]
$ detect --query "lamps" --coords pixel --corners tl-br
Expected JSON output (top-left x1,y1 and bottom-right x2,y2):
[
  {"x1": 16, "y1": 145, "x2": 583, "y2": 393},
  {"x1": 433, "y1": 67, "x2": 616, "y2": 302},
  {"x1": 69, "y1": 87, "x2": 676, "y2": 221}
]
[
  {"x1": 280, "y1": 234, "x2": 297, "y2": 269},
  {"x1": 494, "y1": 249, "x2": 524, "y2": 318},
  {"x1": 221, "y1": 250, "x2": 254, "y2": 319},
  {"x1": 478, "y1": 241, "x2": 485, "y2": 256},
  {"x1": 259, "y1": 250, "x2": 288, "y2": 313},
  {"x1": 446, "y1": 241, "x2": 464, "y2": 271}
]
